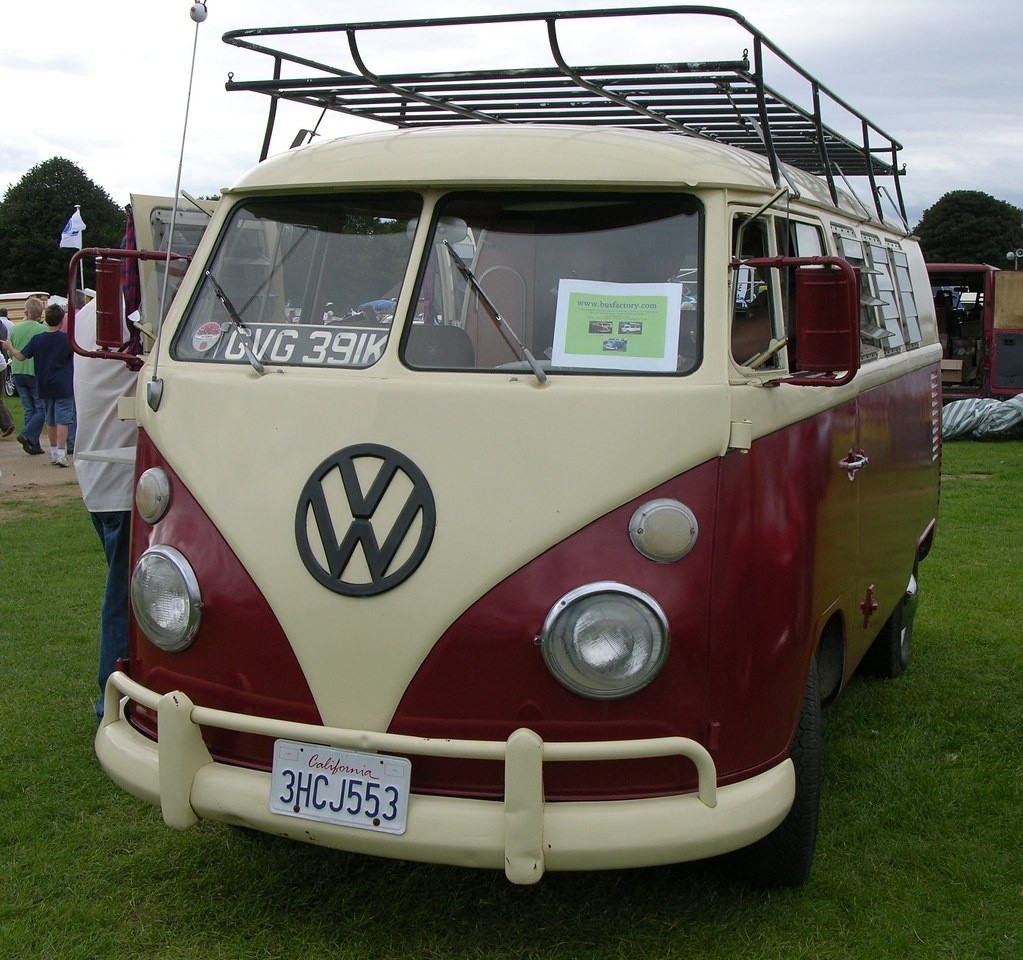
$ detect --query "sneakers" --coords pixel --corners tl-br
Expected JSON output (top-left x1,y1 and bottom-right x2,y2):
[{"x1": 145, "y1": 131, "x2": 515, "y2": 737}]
[
  {"x1": 55, "y1": 456, "x2": 70, "y2": 467},
  {"x1": 48, "y1": 453, "x2": 58, "y2": 463},
  {"x1": 2, "y1": 425, "x2": 15, "y2": 438},
  {"x1": 29, "y1": 447, "x2": 46, "y2": 455},
  {"x1": 16, "y1": 432, "x2": 32, "y2": 453}
]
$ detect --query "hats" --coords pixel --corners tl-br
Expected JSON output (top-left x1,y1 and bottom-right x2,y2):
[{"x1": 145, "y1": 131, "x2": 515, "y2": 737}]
[{"x1": 84, "y1": 289, "x2": 96, "y2": 298}]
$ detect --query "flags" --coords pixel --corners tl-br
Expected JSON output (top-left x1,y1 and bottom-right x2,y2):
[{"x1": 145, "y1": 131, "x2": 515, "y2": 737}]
[{"x1": 60, "y1": 205, "x2": 86, "y2": 250}]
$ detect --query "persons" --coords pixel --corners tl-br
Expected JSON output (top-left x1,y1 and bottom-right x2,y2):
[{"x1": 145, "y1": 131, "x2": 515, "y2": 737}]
[
  {"x1": 0, "y1": 308, "x2": 15, "y2": 341},
  {"x1": 7, "y1": 298, "x2": 46, "y2": 455},
  {"x1": 42, "y1": 295, "x2": 69, "y2": 327},
  {"x1": 83, "y1": 288, "x2": 96, "y2": 304},
  {"x1": 59, "y1": 289, "x2": 85, "y2": 334},
  {"x1": 0, "y1": 302, "x2": 73, "y2": 468},
  {"x1": 0, "y1": 319, "x2": 15, "y2": 438},
  {"x1": 73, "y1": 282, "x2": 145, "y2": 724}
]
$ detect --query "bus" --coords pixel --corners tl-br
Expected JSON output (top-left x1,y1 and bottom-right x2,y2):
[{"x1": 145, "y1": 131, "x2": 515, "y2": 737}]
[{"x1": 68, "y1": 5, "x2": 943, "y2": 885}]
